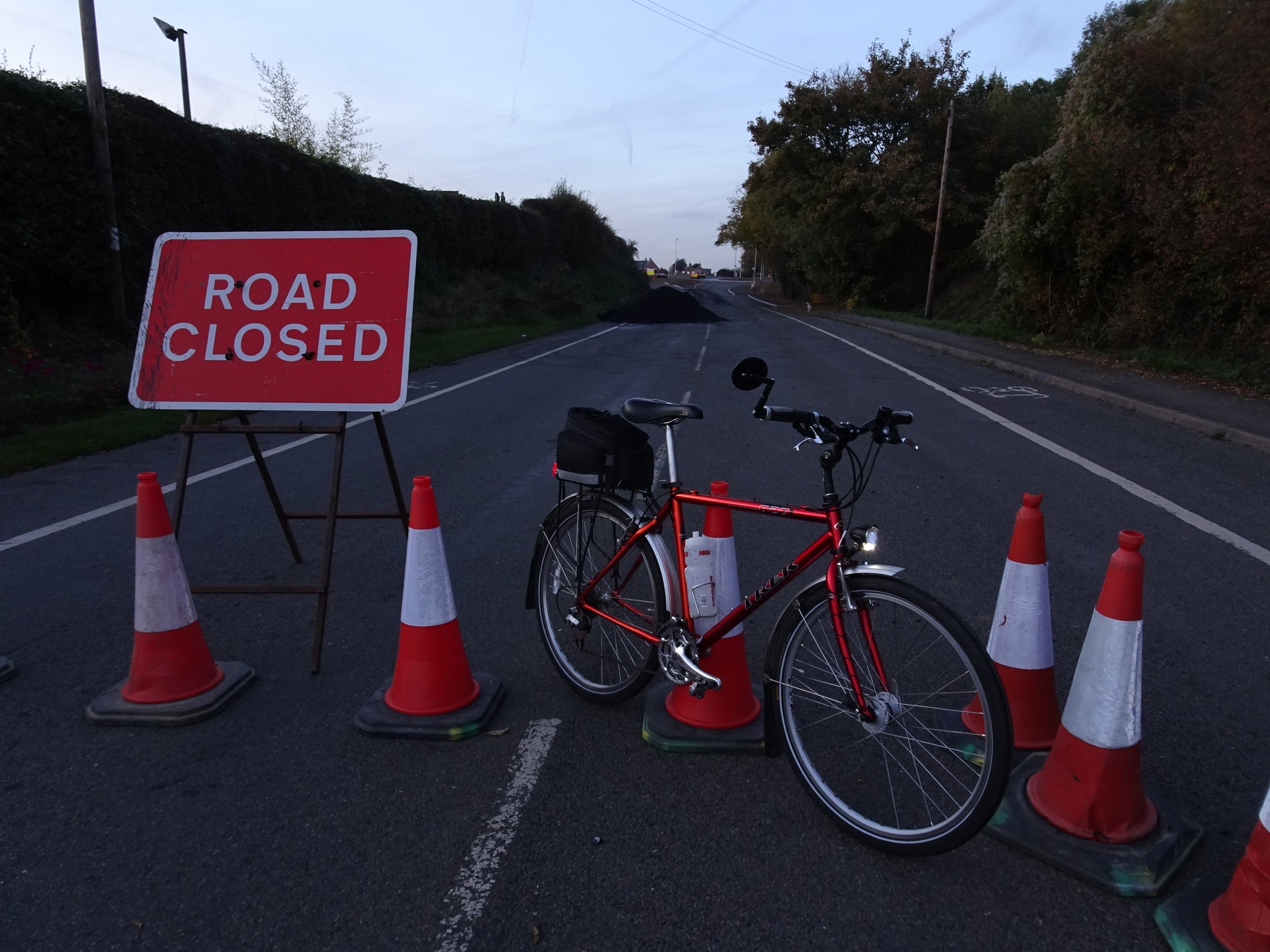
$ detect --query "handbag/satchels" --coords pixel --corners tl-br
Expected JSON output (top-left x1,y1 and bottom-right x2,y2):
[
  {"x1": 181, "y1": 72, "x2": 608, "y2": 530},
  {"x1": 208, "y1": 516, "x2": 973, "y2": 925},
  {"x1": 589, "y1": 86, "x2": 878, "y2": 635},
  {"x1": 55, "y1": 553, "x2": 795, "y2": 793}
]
[{"x1": 558, "y1": 404, "x2": 654, "y2": 490}]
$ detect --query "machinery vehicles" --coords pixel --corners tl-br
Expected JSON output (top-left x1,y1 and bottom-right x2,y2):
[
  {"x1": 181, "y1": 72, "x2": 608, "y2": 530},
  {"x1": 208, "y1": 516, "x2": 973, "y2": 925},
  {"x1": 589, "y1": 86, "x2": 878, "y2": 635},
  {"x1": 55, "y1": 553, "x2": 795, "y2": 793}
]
[{"x1": 646, "y1": 267, "x2": 671, "y2": 278}]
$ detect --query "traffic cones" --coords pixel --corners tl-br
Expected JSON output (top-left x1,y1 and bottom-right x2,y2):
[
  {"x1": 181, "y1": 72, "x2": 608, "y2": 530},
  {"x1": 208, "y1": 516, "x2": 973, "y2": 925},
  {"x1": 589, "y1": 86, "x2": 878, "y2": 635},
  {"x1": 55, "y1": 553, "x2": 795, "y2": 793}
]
[
  {"x1": 935, "y1": 486, "x2": 1060, "y2": 770},
  {"x1": 75, "y1": 466, "x2": 254, "y2": 728},
  {"x1": 628, "y1": 472, "x2": 776, "y2": 746},
  {"x1": 976, "y1": 516, "x2": 1207, "y2": 892},
  {"x1": 338, "y1": 470, "x2": 511, "y2": 741},
  {"x1": 1147, "y1": 763, "x2": 1270, "y2": 951}
]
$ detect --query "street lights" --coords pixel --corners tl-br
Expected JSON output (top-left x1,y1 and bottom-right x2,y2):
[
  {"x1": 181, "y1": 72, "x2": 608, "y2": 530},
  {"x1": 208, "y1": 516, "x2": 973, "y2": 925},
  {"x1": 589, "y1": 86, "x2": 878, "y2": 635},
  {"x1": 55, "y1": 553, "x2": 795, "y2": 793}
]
[{"x1": 673, "y1": 238, "x2": 679, "y2": 277}]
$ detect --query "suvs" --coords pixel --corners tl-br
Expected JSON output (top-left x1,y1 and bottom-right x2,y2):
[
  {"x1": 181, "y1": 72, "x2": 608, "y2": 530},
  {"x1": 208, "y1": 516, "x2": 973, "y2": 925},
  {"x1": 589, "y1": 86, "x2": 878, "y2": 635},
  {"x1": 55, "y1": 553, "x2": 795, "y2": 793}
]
[{"x1": 690, "y1": 270, "x2": 706, "y2": 279}]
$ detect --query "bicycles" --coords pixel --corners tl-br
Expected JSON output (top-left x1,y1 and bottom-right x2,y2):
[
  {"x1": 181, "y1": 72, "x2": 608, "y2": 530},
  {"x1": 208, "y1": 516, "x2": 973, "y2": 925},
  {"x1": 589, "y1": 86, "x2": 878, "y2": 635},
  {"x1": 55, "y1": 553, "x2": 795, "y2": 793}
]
[{"x1": 520, "y1": 347, "x2": 1015, "y2": 855}]
